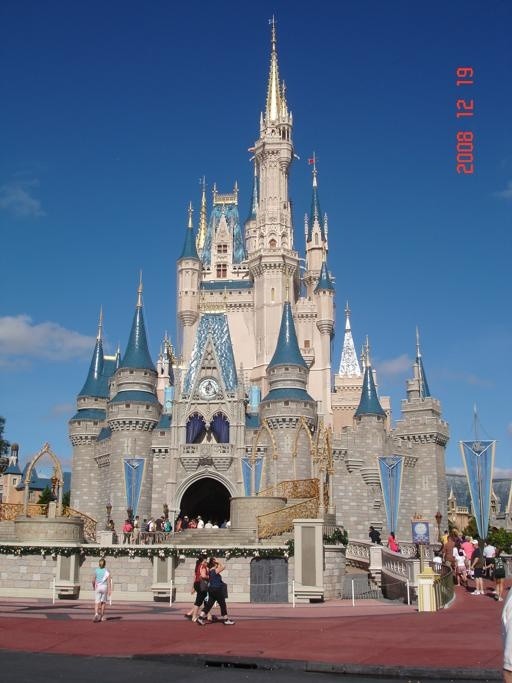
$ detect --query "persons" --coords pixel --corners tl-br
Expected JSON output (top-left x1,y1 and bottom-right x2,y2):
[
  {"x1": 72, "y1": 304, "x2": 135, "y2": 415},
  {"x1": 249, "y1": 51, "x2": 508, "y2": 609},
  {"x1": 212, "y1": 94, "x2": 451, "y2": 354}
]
[
  {"x1": 369, "y1": 526, "x2": 383, "y2": 546},
  {"x1": 436, "y1": 524, "x2": 507, "y2": 603},
  {"x1": 122, "y1": 514, "x2": 173, "y2": 545},
  {"x1": 386, "y1": 531, "x2": 399, "y2": 552},
  {"x1": 190, "y1": 553, "x2": 210, "y2": 621},
  {"x1": 499, "y1": 584, "x2": 511, "y2": 682},
  {"x1": 200, "y1": 557, "x2": 237, "y2": 626},
  {"x1": 90, "y1": 558, "x2": 113, "y2": 623},
  {"x1": 108, "y1": 519, "x2": 117, "y2": 537},
  {"x1": 174, "y1": 512, "x2": 232, "y2": 532},
  {"x1": 185, "y1": 589, "x2": 216, "y2": 619}
]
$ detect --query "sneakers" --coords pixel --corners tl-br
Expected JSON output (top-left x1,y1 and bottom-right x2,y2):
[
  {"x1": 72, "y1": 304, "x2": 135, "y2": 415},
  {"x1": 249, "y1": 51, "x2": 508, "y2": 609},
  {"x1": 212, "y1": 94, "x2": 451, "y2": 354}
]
[
  {"x1": 471, "y1": 589, "x2": 484, "y2": 595},
  {"x1": 93, "y1": 613, "x2": 106, "y2": 622},
  {"x1": 491, "y1": 589, "x2": 503, "y2": 601},
  {"x1": 184, "y1": 613, "x2": 235, "y2": 626}
]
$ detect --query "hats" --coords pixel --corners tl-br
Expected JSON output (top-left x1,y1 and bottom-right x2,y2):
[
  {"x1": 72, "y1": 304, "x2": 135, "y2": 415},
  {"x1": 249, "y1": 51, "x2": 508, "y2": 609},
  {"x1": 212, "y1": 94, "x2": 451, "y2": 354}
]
[{"x1": 472, "y1": 540, "x2": 478, "y2": 543}]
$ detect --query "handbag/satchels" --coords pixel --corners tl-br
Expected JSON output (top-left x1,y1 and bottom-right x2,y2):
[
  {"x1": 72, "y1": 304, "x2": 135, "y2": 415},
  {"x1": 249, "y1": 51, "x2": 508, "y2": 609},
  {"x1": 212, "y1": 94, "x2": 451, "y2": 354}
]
[{"x1": 199, "y1": 575, "x2": 228, "y2": 598}]
